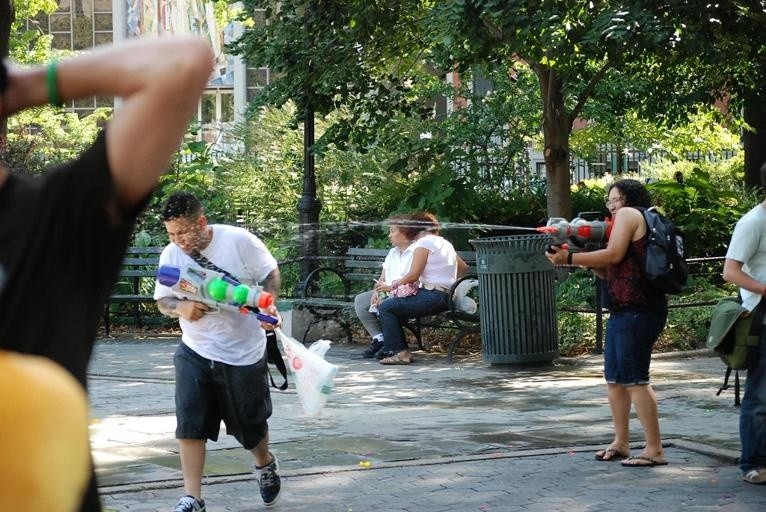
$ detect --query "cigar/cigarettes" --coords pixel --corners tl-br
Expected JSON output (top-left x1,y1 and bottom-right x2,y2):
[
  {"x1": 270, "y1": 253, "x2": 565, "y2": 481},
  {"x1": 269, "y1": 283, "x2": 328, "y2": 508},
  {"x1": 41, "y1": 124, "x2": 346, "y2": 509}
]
[{"x1": 373, "y1": 278, "x2": 379, "y2": 284}]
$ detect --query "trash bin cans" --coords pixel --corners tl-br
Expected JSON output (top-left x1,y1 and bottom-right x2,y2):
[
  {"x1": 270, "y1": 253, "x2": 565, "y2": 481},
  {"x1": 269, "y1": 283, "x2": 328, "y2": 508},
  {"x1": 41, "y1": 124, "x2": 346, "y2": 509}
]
[{"x1": 467, "y1": 234, "x2": 560, "y2": 368}]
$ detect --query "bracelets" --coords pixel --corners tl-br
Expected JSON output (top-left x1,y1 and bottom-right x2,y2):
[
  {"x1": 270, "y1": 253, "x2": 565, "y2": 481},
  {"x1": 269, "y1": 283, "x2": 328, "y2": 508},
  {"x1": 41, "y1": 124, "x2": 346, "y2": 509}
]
[
  {"x1": 586, "y1": 267, "x2": 591, "y2": 271},
  {"x1": 391, "y1": 280, "x2": 398, "y2": 288},
  {"x1": 45, "y1": 55, "x2": 57, "y2": 104},
  {"x1": 567, "y1": 251, "x2": 573, "y2": 267}
]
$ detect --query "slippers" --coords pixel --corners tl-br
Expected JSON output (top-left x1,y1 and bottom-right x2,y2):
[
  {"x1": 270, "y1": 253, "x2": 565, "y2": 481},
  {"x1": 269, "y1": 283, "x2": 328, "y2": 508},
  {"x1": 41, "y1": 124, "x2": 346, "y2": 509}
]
[
  {"x1": 621, "y1": 455, "x2": 668, "y2": 467},
  {"x1": 595, "y1": 447, "x2": 629, "y2": 461},
  {"x1": 380, "y1": 353, "x2": 415, "y2": 365}
]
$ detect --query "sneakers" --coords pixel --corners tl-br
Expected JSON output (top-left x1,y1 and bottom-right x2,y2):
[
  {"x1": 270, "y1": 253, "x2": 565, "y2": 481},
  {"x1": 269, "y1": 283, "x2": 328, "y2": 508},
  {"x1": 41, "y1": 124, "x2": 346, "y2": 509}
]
[
  {"x1": 255, "y1": 450, "x2": 282, "y2": 506},
  {"x1": 363, "y1": 338, "x2": 398, "y2": 359},
  {"x1": 174, "y1": 494, "x2": 206, "y2": 512},
  {"x1": 742, "y1": 468, "x2": 766, "y2": 484}
]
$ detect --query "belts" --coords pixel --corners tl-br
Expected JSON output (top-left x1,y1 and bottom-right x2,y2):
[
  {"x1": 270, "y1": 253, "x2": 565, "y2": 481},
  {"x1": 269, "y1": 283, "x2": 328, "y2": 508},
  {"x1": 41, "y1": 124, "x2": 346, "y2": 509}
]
[{"x1": 424, "y1": 284, "x2": 449, "y2": 294}]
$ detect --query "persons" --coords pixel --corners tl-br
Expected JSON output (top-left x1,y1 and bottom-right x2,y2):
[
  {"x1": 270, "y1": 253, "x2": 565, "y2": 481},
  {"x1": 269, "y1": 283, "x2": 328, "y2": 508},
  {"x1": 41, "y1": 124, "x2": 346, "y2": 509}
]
[
  {"x1": 720, "y1": 158, "x2": 766, "y2": 486},
  {"x1": 0, "y1": 0, "x2": 214, "y2": 512},
  {"x1": 372, "y1": 212, "x2": 471, "y2": 365},
  {"x1": 353, "y1": 211, "x2": 415, "y2": 356},
  {"x1": 544, "y1": 180, "x2": 668, "y2": 467},
  {"x1": 154, "y1": 191, "x2": 282, "y2": 512}
]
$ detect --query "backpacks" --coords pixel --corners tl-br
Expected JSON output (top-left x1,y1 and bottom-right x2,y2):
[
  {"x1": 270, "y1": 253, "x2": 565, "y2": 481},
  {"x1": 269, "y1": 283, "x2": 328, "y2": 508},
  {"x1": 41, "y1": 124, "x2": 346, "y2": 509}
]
[{"x1": 630, "y1": 206, "x2": 688, "y2": 294}]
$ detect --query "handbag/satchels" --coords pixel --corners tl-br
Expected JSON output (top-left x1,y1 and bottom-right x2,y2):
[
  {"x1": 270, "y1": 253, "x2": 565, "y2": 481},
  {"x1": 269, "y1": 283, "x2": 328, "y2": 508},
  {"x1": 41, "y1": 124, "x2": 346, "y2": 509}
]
[
  {"x1": 706, "y1": 297, "x2": 761, "y2": 370},
  {"x1": 390, "y1": 279, "x2": 420, "y2": 298}
]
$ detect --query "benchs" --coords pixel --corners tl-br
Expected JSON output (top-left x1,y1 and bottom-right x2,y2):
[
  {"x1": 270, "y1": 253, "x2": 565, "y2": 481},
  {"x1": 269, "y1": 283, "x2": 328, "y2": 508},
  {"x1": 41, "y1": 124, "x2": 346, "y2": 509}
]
[
  {"x1": 274, "y1": 247, "x2": 481, "y2": 363},
  {"x1": 99, "y1": 245, "x2": 163, "y2": 338}
]
